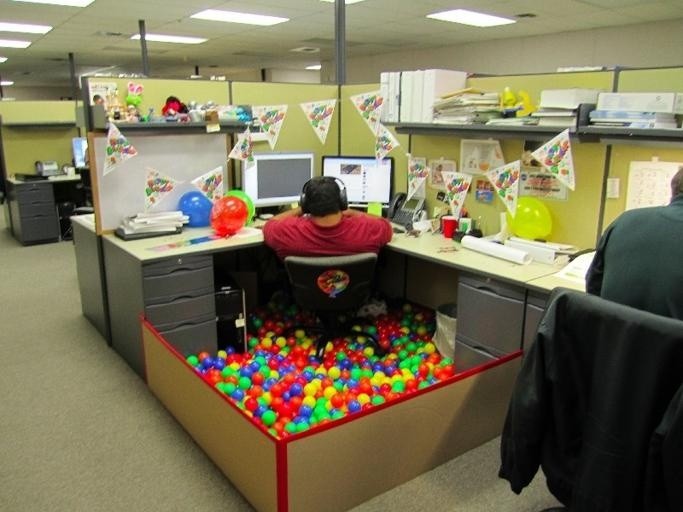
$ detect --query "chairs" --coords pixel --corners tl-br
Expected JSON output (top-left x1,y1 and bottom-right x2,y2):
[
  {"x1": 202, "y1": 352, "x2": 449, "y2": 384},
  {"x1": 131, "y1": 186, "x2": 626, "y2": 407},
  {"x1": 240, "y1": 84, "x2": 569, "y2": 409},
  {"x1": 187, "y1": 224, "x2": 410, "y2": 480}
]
[{"x1": 526, "y1": 288, "x2": 683, "y2": 512}]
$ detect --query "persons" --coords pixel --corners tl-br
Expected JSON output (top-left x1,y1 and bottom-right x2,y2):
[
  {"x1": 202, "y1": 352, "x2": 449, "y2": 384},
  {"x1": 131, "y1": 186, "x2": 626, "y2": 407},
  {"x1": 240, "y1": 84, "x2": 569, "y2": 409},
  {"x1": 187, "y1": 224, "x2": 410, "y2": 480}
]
[
  {"x1": 584, "y1": 166, "x2": 683, "y2": 322},
  {"x1": 263, "y1": 175, "x2": 392, "y2": 263}
]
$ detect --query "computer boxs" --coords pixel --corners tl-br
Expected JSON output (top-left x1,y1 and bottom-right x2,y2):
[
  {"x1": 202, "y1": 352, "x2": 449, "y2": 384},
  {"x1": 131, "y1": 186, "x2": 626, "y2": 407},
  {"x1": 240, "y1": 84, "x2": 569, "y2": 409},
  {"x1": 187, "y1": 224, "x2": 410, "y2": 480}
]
[
  {"x1": 215, "y1": 274, "x2": 247, "y2": 355},
  {"x1": 56, "y1": 201, "x2": 76, "y2": 241}
]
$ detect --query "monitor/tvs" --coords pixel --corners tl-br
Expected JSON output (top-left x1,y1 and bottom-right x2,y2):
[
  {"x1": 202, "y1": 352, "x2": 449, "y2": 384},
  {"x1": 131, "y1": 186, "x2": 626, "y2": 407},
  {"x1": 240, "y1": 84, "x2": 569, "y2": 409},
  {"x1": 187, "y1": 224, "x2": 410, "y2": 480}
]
[
  {"x1": 73, "y1": 137, "x2": 88, "y2": 169},
  {"x1": 321, "y1": 155, "x2": 394, "y2": 212},
  {"x1": 242, "y1": 153, "x2": 314, "y2": 218}
]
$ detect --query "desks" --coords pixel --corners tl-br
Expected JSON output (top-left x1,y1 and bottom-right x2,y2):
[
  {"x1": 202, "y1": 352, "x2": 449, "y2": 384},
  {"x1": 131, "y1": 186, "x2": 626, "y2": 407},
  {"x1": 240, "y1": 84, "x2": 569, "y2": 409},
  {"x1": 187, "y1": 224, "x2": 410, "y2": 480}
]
[{"x1": 7, "y1": 172, "x2": 596, "y2": 372}]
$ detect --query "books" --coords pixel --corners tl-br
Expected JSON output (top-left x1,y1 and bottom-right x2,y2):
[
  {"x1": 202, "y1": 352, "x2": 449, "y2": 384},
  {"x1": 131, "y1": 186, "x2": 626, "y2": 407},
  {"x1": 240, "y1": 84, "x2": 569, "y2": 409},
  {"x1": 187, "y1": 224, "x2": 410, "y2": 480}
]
[
  {"x1": 432, "y1": 85, "x2": 679, "y2": 132},
  {"x1": 113, "y1": 210, "x2": 190, "y2": 241}
]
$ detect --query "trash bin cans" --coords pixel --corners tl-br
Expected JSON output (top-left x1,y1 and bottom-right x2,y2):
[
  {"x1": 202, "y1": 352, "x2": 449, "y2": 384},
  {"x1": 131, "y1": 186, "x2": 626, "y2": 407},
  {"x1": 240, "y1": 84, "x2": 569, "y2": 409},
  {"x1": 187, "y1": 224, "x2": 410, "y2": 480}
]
[{"x1": 432, "y1": 303, "x2": 457, "y2": 362}]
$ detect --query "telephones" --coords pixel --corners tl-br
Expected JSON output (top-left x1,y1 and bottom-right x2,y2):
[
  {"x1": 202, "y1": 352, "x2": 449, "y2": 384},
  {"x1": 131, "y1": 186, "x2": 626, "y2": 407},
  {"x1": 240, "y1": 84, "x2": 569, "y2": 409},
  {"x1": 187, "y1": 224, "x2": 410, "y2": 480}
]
[
  {"x1": 388, "y1": 193, "x2": 425, "y2": 226},
  {"x1": 35, "y1": 161, "x2": 58, "y2": 178}
]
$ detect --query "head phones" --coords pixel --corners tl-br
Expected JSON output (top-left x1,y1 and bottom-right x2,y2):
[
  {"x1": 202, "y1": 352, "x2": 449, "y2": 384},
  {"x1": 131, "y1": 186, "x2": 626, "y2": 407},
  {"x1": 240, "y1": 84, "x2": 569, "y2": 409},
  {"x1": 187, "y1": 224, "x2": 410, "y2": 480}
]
[{"x1": 300, "y1": 176, "x2": 348, "y2": 213}]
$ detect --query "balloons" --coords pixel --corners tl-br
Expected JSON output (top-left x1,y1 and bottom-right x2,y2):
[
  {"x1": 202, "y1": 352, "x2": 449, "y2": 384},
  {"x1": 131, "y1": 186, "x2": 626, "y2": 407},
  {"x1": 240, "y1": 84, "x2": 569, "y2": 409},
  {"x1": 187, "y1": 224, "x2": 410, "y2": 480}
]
[
  {"x1": 506, "y1": 197, "x2": 552, "y2": 240},
  {"x1": 208, "y1": 196, "x2": 248, "y2": 239},
  {"x1": 176, "y1": 191, "x2": 212, "y2": 227},
  {"x1": 223, "y1": 190, "x2": 255, "y2": 228}
]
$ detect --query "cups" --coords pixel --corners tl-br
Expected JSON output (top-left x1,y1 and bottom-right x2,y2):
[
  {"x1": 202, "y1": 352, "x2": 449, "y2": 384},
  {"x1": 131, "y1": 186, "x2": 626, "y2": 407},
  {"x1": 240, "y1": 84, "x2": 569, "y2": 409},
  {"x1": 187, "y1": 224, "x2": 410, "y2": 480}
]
[{"x1": 442, "y1": 216, "x2": 457, "y2": 238}]
